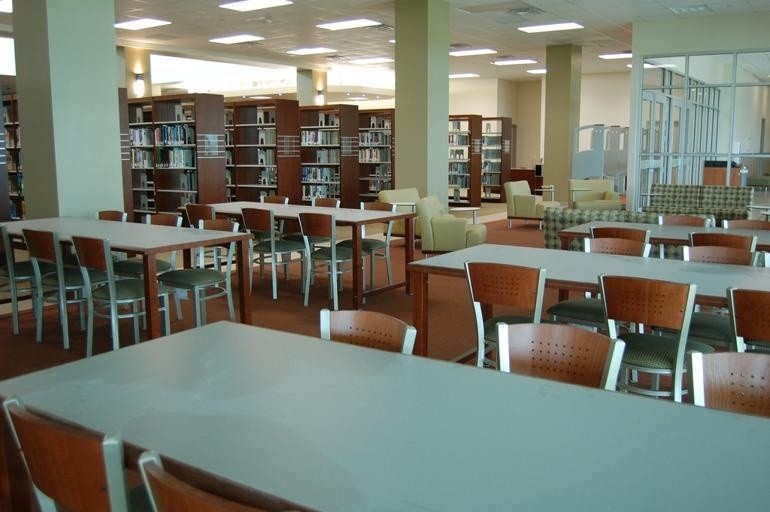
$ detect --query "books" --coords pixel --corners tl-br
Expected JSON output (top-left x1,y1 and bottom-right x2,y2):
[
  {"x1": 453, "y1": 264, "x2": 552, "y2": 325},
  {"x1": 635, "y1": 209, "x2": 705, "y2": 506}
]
[
  {"x1": 358, "y1": 132, "x2": 391, "y2": 191},
  {"x1": 300, "y1": 130, "x2": 341, "y2": 199},
  {"x1": 130, "y1": 125, "x2": 197, "y2": 204},
  {"x1": 264, "y1": 129, "x2": 277, "y2": 185},
  {"x1": 3, "y1": 105, "x2": 24, "y2": 220},
  {"x1": 481, "y1": 136, "x2": 502, "y2": 185},
  {"x1": 449, "y1": 134, "x2": 470, "y2": 188},
  {"x1": 225, "y1": 130, "x2": 234, "y2": 198}
]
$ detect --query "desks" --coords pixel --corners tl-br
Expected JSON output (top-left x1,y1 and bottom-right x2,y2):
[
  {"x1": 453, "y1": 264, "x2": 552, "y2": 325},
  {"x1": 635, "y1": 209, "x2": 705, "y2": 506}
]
[{"x1": 704, "y1": 160, "x2": 749, "y2": 187}]
